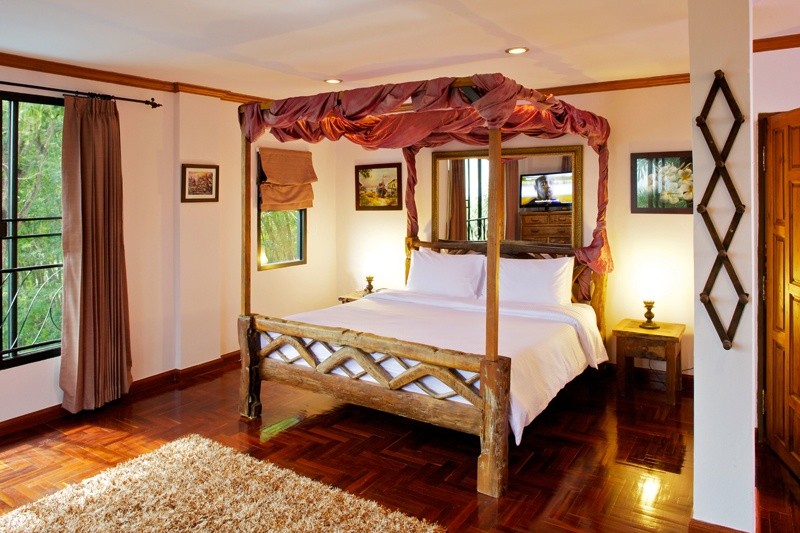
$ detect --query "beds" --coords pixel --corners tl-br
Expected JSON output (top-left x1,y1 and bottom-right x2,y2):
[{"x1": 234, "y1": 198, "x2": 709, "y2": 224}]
[{"x1": 235, "y1": 72, "x2": 615, "y2": 500}]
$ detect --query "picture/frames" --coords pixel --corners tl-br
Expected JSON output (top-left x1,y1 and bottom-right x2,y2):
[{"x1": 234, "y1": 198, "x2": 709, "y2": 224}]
[
  {"x1": 630, "y1": 150, "x2": 694, "y2": 214},
  {"x1": 353, "y1": 161, "x2": 403, "y2": 211},
  {"x1": 181, "y1": 163, "x2": 220, "y2": 203}
]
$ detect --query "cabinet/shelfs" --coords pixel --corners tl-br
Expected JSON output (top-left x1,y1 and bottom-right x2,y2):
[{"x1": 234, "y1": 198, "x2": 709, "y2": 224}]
[{"x1": 518, "y1": 210, "x2": 572, "y2": 244}]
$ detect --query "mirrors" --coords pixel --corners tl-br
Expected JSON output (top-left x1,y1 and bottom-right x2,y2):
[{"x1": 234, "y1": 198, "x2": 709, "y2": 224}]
[{"x1": 431, "y1": 145, "x2": 584, "y2": 253}]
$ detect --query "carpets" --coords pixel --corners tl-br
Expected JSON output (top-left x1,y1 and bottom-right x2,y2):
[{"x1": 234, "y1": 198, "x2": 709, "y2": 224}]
[{"x1": 0, "y1": 433, "x2": 449, "y2": 533}]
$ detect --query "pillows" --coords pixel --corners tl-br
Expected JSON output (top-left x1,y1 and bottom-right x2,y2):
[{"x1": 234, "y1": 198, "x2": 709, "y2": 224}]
[
  {"x1": 405, "y1": 249, "x2": 486, "y2": 300},
  {"x1": 479, "y1": 255, "x2": 576, "y2": 305},
  {"x1": 418, "y1": 245, "x2": 485, "y2": 299}
]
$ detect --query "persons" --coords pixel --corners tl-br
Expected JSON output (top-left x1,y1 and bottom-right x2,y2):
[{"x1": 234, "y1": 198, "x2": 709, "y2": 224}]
[{"x1": 528, "y1": 176, "x2": 561, "y2": 205}]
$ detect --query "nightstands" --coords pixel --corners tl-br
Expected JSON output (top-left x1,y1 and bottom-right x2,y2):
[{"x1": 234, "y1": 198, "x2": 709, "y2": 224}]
[
  {"x1": 611, "y1": 319, "x2": 686, "y2": 408},
  {"x1": 336, "y1": 287, "x2": 383, "y2": 305}
]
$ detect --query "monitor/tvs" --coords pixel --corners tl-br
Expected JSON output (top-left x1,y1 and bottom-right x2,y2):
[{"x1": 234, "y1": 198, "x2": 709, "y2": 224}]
[{"x1": 517, "y1": 171, "x2": 573, "y2": 213}]
